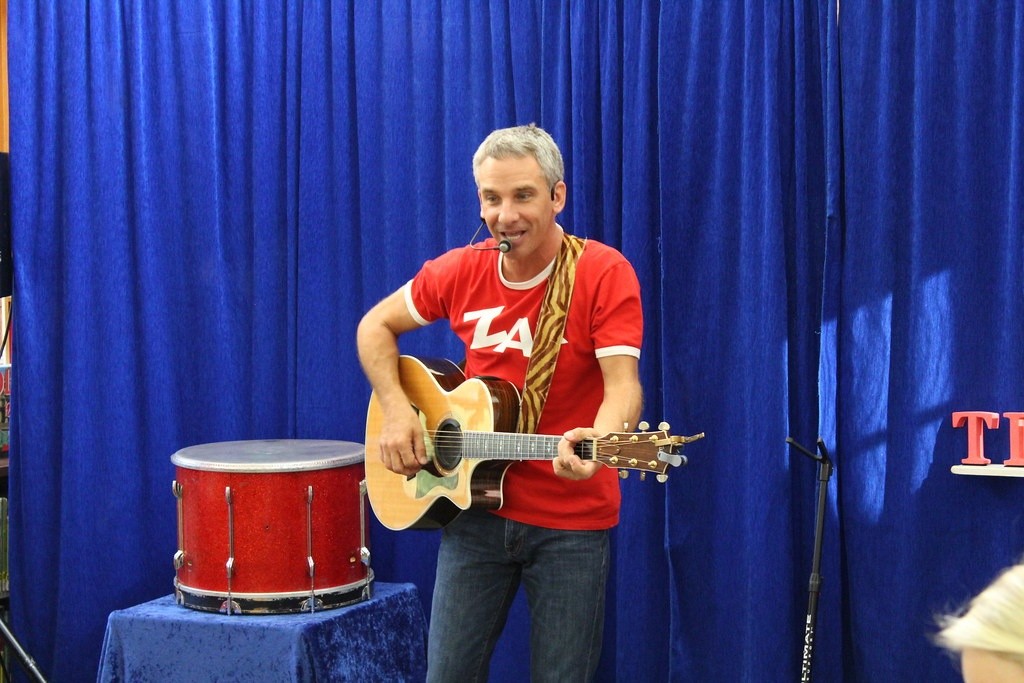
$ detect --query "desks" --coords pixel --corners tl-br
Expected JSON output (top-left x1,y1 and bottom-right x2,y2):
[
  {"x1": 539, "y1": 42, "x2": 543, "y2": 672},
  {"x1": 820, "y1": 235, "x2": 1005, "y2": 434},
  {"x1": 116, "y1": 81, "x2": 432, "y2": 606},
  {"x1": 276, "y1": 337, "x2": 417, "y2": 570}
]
[{"x1": 96, "y1": 582, "x2": 427, "y2": 683}]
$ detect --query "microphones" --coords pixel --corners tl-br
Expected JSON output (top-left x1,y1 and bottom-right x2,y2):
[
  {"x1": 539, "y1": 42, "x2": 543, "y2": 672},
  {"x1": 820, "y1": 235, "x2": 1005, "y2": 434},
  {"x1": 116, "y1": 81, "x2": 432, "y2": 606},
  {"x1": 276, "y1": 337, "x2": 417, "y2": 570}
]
[{"x1": 470, "y1": 217, "x2": 512, "y2": 253}]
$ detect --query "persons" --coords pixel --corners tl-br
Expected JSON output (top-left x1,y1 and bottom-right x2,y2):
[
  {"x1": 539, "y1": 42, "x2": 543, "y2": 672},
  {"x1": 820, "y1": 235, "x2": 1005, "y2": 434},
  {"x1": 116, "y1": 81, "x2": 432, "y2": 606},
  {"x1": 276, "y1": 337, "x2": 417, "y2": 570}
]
[{"x1": 356, "y1": 123, "x2": 646, "y2": 683}]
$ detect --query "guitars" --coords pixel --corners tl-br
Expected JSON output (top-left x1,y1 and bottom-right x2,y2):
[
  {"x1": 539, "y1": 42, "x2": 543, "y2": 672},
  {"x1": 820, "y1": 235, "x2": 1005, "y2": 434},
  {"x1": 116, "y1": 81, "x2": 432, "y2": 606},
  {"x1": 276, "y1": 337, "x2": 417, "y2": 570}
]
[{"x1": 364, "y1": 353, "x2": 708, "y2": 537}]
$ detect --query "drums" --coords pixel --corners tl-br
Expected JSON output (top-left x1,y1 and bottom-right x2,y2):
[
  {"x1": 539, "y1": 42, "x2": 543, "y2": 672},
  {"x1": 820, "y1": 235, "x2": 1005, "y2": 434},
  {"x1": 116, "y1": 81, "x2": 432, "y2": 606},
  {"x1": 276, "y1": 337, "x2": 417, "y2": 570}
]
[{"x1": 167, "y1": 437, "x2": 375, "y2": 616}]
